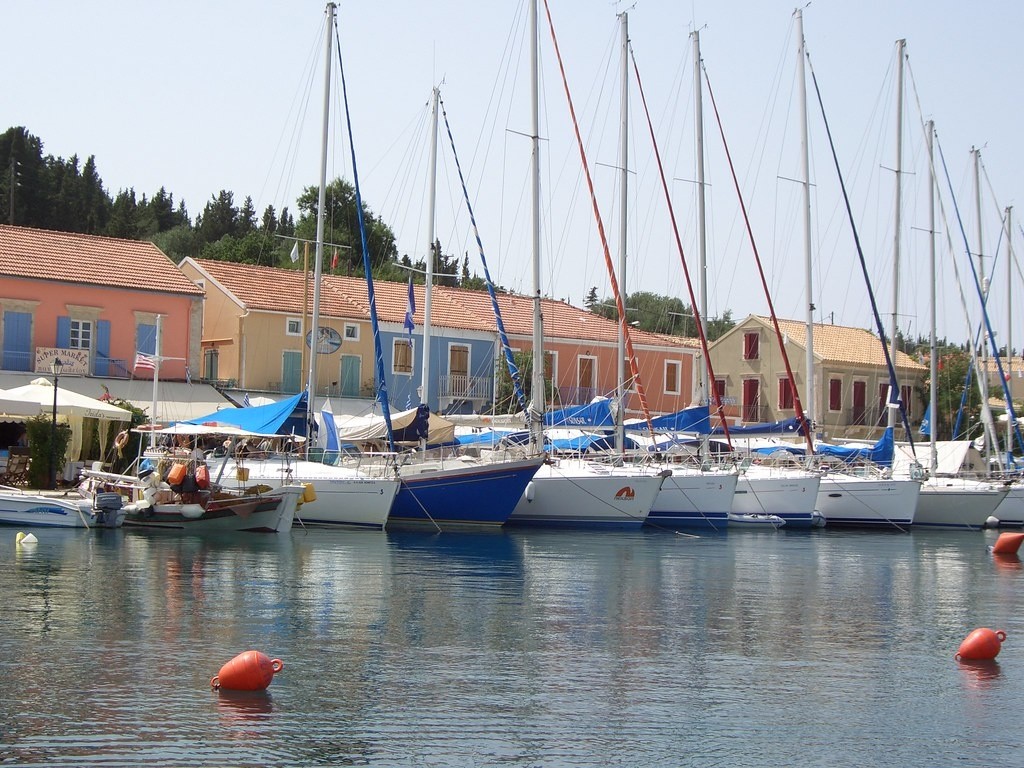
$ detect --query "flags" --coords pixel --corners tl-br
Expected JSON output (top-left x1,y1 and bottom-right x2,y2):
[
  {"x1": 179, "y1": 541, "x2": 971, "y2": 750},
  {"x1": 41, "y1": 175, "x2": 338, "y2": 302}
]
[
  {"x1": 403, "y1": 274, "x2": 416, "y2": 349},
  {"x1": 244, "y1": 393, "x2": 250, "y2": 407},
  {"x1": 186, "y1": 366, "x2": 193, "y2": 389},
  {"x1": 134, "y1": 350, "x2": 157, "y2": 369},
  {"x1": 939, "y1": 349, "x2": 943, "y2": 369},
  {"x1": 332, "y1": 247, "x2": 338, "y2": 268},
  {"x1": 919, "y1": 350, "x2": 925, "y2": 366},
  {"x1": 290, "y1": 240, "x2": 299, "y2": 263}
]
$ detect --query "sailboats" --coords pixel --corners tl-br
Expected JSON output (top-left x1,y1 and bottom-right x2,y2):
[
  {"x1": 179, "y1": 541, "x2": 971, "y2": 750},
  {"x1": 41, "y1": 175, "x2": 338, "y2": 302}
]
[{"x1": 138, "y1": 2, "x2": 1024, "y2": 531}]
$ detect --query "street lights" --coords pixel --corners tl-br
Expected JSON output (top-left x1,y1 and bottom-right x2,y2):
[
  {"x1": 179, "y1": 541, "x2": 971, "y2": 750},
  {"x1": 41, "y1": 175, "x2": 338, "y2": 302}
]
[{"x1": 41, "y1": 357, "x2": 64, "y2": 489}]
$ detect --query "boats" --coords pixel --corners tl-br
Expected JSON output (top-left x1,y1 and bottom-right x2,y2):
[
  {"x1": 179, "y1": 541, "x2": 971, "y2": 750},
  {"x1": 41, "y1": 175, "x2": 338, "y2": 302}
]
[
  {"x1": 0, "y1": 483, "x2": 128, "y2": 529},
  {"x1": 75, "y1": 419, "x2": 306, "y2": 535}
]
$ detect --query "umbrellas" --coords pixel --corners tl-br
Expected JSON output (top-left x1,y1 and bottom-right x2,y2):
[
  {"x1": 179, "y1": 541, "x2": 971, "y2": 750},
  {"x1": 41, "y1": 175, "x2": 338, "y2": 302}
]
[{"x1": 0, "y1": 378, "x2": 132, "y2": 431}]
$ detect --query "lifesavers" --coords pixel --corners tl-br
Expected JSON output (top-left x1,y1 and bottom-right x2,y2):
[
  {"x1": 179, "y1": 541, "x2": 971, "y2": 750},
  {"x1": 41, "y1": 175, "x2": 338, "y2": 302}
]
[
  {"x1": 176, "y1": 434, "x2": 190, "y2": 448},
  {"x1": 114, "y1": 430, "x2": 129, "y2": 449}
]
